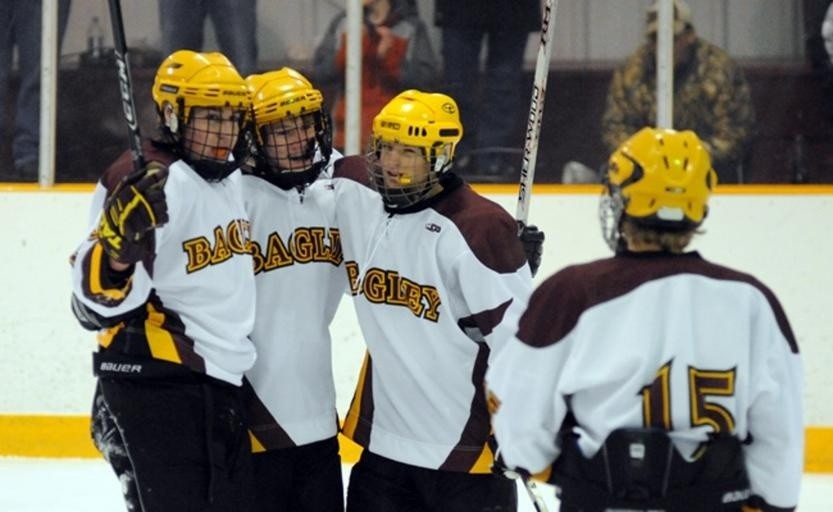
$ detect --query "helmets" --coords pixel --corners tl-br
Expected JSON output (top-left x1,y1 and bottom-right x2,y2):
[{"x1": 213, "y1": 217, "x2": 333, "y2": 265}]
[
  {"x1": 242, "y1": 68, "x2": 329, "y2": 179},
  {"x1": 373, "y1": 90, "x2": 464, "y2": 205},
  {"x1": 610, "y1": 127, "x2": 718, "y2": 235},
  {"x1": 152, "y1": 49, "x2": 249, "y2": 179}
]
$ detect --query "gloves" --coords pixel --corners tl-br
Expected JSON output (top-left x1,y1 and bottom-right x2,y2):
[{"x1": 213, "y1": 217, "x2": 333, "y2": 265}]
[
  {"x1": 517, "y1": 221, "x2": 544, "y2": 277},
  {"x1": 95, "y1": 167, "x2": 170, "y2": 264}
]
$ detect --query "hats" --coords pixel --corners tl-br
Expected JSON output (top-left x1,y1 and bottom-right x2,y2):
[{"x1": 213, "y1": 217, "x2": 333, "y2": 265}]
[{"x1": 643, "y1": 2, "x2": 692, "y2": 39}]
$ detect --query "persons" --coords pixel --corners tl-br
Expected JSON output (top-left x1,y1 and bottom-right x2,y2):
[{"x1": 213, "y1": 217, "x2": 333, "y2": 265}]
[
  {"x1": 482, "y1": 125, "x2": 804, "y2": 510},
  {"x1": 67, "y1": 48, "x2": 259, "y2": 510},
  {"x1": 432, "y1": 1, "x2": 546, "y2": 174},
  {"x1": 2, "y1": 0, "x2": 72, "y2": 181},
  {"x1": 158, "y1": 1, "x2": 258, "y2": 78},
  {"x1": 308, "y1": 0, "x2": 437, "y2": 157},
  {"x1": 597, "y1": 1, "x2": 757, "y2": 183},
  {"x1": 315, "y1": 87, "x2": 536, "y2": 511},
  {"x1": 239, "y1": 66, "x2": 547, "y2": 512}
]
